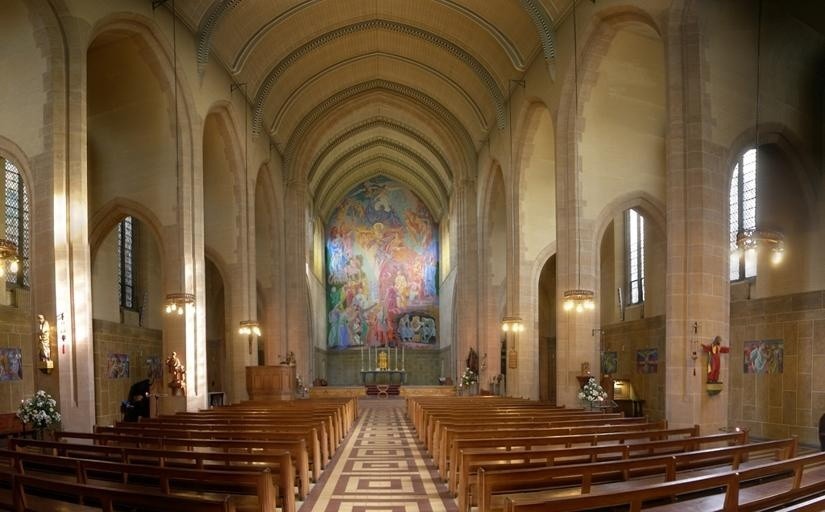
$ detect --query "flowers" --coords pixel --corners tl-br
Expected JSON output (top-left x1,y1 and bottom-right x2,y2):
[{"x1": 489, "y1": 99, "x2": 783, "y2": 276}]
[
  {"x1": 458, "y1": 368, "x2": 477, "y2": 387},
  {"x1": 294, "y1": 374, "x2": 308, "y2": 390},
  {"x1": 19, "y1": 388, "x2": 62, "y2": 426},
  {"x1": 579, "y1": 377, "x2": 606, "y2": 401}
]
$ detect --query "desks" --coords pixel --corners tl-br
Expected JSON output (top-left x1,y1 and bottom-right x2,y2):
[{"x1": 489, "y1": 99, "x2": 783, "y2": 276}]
[{"x1": 360, "y1": 371, "x2": 404, "y2": 385}]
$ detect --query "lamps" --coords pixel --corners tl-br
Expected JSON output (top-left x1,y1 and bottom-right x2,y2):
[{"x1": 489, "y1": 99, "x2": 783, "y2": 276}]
[
  {"x1": 0, "y1": 238, "x2": 20, "y2": 277},
  {"x1": 736, "y1": 229, "x2": 787, "y2": 264},
  {"x1": 562, "y1": 290, "x2": 597, "y2": 314},
  {"x1": 502, "y1": 316, "x2": 525, "y2": 333},
  {"x1": 238, "y1": 320, "x2": 264, "y2": 337},
  {"x1": 166, "y1": 292, "x2": 199, "y2": 315}
]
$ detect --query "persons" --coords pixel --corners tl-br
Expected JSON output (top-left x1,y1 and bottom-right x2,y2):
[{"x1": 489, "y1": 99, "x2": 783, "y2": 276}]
[
  {"x1": 165, "y1": 351, "x2": 181, "y2": 383},
  {"x1": 744, "y1": 341, "x2": 783, "y2": 374},
  {"x1": 37, "y1": 314, "x2": 51, "y2": 361},
  {"x1": 326, "y1": 181, "x2": 438, "y2": 351},
  {"x1": 700, "y1": 334, "x2": 732, "y2": 383}
]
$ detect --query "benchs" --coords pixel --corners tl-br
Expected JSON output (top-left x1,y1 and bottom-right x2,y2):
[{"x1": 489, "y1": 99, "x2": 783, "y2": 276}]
[
  {"x1": 405, "y1": 392, "x2": 824, "y2": 512},
  {"x1": 0, "y1": 394, "x2": 359, "y2": 511}
]
